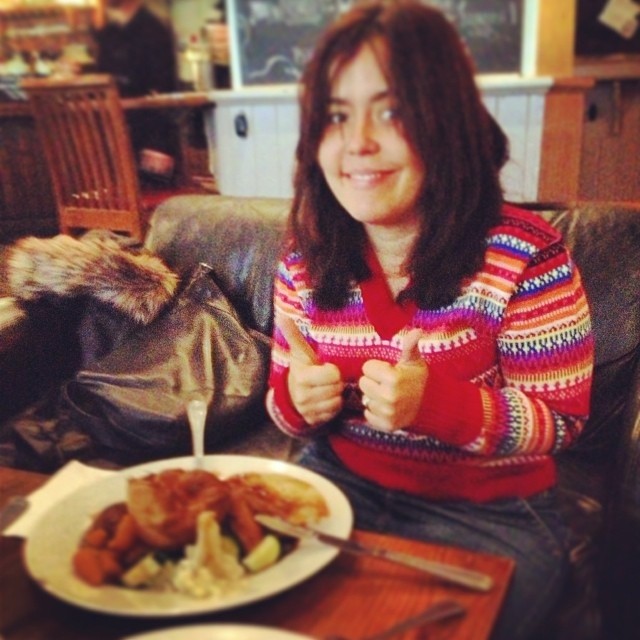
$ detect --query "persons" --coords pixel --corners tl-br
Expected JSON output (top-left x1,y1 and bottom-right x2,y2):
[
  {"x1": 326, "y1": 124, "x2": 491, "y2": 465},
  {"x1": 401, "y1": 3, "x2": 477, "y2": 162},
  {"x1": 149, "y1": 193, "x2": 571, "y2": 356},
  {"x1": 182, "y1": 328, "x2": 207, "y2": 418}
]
[
  {"x1": 61, "y1": 1, "x2": 180, "y2": 188},
  {"x1": 259, "y1": 0, "x2": 597, "y2": 640}
]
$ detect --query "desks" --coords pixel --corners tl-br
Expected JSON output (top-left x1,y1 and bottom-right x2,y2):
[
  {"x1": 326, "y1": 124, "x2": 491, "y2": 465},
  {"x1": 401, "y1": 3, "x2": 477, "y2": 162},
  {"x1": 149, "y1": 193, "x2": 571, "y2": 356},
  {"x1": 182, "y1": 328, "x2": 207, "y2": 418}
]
[{"x1": 1, "y1": 92, "x2": 212, "y2": 240}]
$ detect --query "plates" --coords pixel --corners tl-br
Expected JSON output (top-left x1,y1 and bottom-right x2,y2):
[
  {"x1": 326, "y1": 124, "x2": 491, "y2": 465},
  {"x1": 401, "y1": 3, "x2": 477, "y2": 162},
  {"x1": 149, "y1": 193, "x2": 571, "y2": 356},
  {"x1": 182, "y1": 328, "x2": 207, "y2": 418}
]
[
  {"x1": 23, "y1": 455, "x2": 353, "y2": 617},
  {"x1": 121, "y1": 623, "x2": 317, "y2": 640}
]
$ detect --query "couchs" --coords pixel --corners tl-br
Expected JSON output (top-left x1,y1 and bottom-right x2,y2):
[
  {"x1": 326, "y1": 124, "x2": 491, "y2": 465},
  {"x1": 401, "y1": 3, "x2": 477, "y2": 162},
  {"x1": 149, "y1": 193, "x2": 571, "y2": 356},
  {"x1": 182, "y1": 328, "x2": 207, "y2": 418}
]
[{"x1": 0, "y1": 193, "x2": 639, "y2": 639}]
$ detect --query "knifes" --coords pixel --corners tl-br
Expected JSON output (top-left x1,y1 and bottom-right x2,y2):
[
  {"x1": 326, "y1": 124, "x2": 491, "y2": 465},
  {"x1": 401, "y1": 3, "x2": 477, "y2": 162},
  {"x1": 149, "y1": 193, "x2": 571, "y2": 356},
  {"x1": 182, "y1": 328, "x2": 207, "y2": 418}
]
[{"x1": 255, "y1": 515, "x2": 492, "y2": 593}]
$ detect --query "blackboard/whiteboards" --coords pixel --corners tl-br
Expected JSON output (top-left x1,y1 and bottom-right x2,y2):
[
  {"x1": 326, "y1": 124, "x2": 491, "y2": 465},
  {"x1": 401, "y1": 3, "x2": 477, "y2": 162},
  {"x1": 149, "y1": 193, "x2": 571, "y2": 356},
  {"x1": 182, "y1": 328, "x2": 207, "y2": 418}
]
[{"x1": 228, "y1": 0, "x2": 538, "y2": 90}]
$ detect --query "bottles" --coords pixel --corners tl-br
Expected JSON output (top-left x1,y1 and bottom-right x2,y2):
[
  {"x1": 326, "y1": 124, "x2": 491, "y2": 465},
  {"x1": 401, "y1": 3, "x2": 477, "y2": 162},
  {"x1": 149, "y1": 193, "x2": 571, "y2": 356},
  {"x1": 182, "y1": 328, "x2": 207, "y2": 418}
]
[{"x1": 187, "y1": 36, "x2": 213, "y2": 93}]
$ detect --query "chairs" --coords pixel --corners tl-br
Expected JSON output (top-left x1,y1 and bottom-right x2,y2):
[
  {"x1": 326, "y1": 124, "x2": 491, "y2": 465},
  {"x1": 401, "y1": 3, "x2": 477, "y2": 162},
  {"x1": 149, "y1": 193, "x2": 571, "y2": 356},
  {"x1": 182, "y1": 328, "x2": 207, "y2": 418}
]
[{"x1": 17, "y1": 74, "x2": 221, "y2": 243}]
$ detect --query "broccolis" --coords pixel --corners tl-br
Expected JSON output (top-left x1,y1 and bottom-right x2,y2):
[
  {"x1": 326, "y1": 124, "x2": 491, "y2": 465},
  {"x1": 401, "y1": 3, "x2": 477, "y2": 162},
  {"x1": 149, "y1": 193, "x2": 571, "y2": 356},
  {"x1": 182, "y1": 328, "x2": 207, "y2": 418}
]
[{"x1": 172, "y1": 511, "x2": 243, "y2": 602}]
